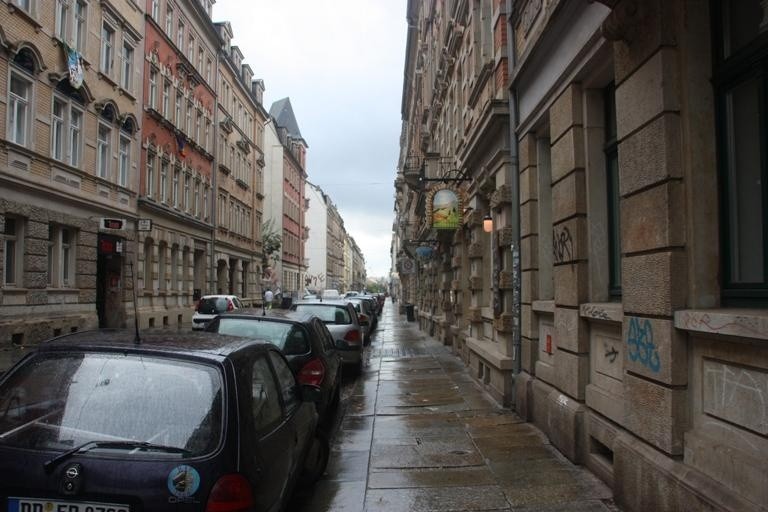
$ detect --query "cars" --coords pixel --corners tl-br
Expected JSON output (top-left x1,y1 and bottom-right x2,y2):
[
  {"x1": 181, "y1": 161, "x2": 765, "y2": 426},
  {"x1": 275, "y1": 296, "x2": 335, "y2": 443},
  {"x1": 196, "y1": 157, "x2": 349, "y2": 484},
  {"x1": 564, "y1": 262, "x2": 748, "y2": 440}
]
[
  {"x1": 191, "y1": 285, "x2": 387, "y2": 433},
  {"x1": 0, "y1": 326, "x2": 332, "y2": 512}
]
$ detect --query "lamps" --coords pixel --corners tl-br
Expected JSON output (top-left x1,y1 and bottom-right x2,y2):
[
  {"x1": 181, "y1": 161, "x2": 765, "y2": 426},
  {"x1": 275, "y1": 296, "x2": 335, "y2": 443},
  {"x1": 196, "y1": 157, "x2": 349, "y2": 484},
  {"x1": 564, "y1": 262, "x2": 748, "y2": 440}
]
[{"x1": 483, "y1": 205, "x2": 501, "y2": 233}]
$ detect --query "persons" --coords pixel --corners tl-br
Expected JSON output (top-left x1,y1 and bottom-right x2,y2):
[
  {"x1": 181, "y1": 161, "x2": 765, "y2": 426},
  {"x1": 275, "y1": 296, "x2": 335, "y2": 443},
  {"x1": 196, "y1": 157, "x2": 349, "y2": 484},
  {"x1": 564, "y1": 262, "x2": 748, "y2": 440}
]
[{"x1": 264, "y1": 288, "x2": 274, "y2": 310}]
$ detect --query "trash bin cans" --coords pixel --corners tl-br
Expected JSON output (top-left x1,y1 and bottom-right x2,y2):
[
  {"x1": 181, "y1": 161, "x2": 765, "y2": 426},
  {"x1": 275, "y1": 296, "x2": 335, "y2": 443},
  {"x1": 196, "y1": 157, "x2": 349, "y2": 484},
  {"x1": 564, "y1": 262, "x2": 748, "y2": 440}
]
[{"x1": 405, "y1": 304, "x2": 414, "y2": 321}]
